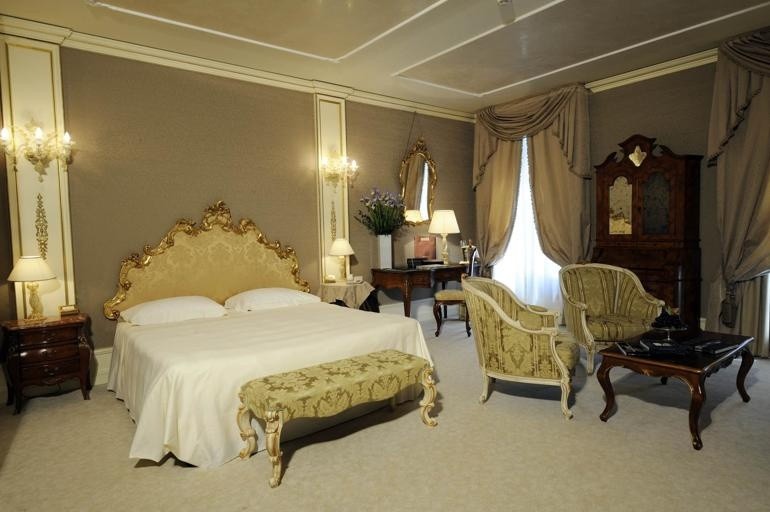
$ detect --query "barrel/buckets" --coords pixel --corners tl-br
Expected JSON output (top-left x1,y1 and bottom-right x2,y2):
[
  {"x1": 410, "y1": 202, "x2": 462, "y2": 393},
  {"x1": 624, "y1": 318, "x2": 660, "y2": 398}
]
[{"x1": 462, "y1": 245, "x2": 476, "y2": 261}]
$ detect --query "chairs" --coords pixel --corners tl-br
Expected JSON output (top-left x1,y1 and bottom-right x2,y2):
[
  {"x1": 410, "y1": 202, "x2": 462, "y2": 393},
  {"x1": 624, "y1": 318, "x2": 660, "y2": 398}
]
[
  {"x1": 559, "y1": 262, "x2": 666, "y2": 376},
  {"x1": 460, "y1": 272, "x2": 581, "y2": 421},
  {"x1": 433, "y1": 246, "x2": 477, "y2": 338}
]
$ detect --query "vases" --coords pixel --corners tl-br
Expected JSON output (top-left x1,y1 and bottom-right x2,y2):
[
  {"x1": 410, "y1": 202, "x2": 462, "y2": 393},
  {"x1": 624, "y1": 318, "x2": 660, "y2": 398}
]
[{"x1": 373, "y1": 233, "x2": 392, "y2": 270}]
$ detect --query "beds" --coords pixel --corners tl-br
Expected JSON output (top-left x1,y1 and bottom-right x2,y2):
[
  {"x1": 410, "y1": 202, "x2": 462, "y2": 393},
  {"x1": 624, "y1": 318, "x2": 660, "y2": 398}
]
[
  {"x1": 102, "y1": 200, "x2": 434, "y2": 468},
  {"x1": 609, "y1": 211, "x2": 632, "y2": 234}
]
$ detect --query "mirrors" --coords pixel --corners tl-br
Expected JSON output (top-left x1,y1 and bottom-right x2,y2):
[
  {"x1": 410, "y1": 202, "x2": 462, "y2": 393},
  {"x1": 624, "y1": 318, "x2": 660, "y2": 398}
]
[
  {"x1": 607, "y1": 175, "x2": 632, "y2": 234},
  {"x1": 398, "y1": 110, "x2": 439, "y2": 228}
]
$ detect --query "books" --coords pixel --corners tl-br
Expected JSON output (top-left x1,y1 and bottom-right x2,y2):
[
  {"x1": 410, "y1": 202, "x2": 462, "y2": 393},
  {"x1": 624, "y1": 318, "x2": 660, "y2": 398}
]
[
  {"x1": 615, "y1": 340, "x2": 650, "y2": 357},
  {"x1": 640, "y1": 337, "x2": 680, "y2": 352},
  {"x1": 695, "y1": 338, "x2": 739, "y2": 354}
]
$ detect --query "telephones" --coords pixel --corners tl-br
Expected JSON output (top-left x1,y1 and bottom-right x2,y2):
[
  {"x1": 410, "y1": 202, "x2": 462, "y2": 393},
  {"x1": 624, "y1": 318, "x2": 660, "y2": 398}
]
[{"x1": 348, "y1": 274, "x2": 364, "y2": 284}]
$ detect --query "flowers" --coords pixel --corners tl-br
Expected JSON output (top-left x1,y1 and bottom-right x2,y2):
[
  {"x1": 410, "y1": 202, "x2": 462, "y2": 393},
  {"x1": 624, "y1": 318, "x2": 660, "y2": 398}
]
[{"x1": 353, "y1": 186, "x2": 416, "y2": 235}]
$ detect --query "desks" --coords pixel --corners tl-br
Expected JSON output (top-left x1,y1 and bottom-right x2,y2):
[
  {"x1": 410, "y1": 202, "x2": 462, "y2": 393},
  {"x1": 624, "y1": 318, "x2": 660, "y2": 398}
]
[{"x1": 371, "y1": 262, "x2": 470, "y2": 318}]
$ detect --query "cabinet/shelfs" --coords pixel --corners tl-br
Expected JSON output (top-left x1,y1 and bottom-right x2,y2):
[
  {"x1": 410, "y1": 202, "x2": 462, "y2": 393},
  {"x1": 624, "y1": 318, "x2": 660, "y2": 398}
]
[{"x1": 592, "y1": 131, "x2": 704, "y2": 332}]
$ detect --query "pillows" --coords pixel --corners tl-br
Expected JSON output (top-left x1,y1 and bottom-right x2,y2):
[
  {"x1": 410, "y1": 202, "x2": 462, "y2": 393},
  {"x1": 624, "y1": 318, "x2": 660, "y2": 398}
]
[
  {"x1": 224, "y1": 287, "x2": 320, "y2": 313},
  {"x1": 118, "y1": 295, "x2": 228, "y2": 325}
]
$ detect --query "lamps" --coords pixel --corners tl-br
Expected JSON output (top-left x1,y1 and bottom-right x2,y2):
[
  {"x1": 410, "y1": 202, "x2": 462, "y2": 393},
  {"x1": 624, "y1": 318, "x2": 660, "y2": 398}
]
[
  {"x1": 0, "y1": 120, "x2": 77, "y2": 182},
  {"x1": 329, "y1": 239, "x2": 355, "y2": 285},
  {"x1": 497, "y1": 0, "x2": 515, "y2": 24},
  {"x1": 406, "y1": 209, "x2": 422, "y2": 222},
  {"x1": 322, "y1": 152, "x2": 359, "y2": 190},
  {"x1": 428, "y1": 209, "x2": 461, "y2": 265},
  {"x1": 7, "y1": 255, "x2": 56, "y2": 319}
]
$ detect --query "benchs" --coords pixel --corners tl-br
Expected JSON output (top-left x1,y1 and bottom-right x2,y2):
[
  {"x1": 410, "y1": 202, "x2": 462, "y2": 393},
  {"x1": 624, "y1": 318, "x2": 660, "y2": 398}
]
[{"x1": 237, "y1": 348, "x2": 438, "y2": 487}]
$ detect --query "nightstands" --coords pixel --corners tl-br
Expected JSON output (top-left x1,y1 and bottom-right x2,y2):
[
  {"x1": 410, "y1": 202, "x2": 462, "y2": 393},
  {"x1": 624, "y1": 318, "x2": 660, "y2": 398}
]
[{"x1": 0, "y1": 312, "x2": 93, "y2": 415}]
